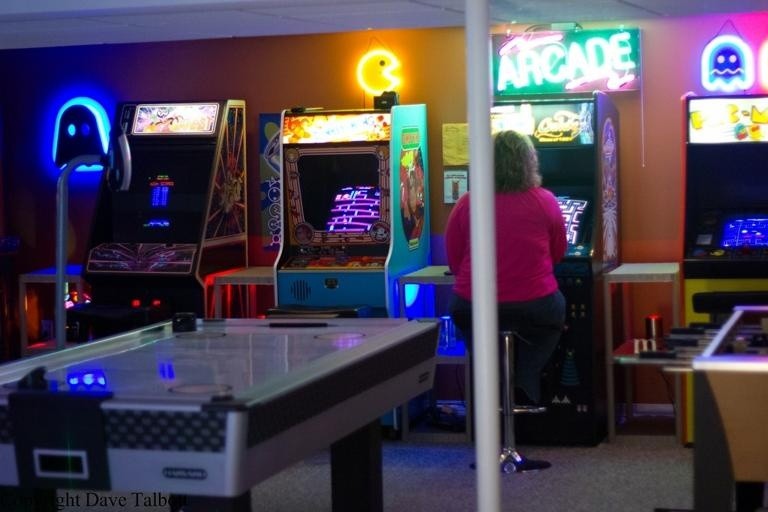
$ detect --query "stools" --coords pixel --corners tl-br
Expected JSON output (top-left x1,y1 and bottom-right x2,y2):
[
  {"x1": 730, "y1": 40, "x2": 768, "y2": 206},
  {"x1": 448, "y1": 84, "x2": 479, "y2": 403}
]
[{"x1": 469, "y1": 324, "x2": 554, "y2": 473}]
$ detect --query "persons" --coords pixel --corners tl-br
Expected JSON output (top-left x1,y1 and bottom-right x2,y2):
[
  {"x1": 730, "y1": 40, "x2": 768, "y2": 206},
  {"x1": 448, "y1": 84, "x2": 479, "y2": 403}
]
[{"x1": 443, "y1": 128, "x2": 568, "y2": 410}]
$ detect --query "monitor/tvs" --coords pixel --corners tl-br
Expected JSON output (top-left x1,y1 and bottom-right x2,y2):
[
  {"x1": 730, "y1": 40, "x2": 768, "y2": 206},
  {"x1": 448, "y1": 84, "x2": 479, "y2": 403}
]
[
  {"x1": 687, "y1": 143, "x2": 768, "y2": 250},
  {"x1": 537, "y1": 146, "x2": 593, "y2": 246},
  {"x1": 284, "y1": 142, "x2": 389, "y2": 247},
  {"x1": 108, "y1": 144, "x2": 215, "y2": 243}
]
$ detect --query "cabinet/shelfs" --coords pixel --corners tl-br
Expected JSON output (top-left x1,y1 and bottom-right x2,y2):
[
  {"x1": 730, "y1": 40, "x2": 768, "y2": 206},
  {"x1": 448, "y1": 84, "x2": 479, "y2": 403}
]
[
  {"x1": 20, "y1": 264, "x2": 86, "y2": 361},
  {"x1": 601, "y1": 259, "x2": 682, "y2": 446},
  {"x1": 398, "y1": 264, "x2": 474, "y2": 445},
  {"x1": 212, "y1": 266, "x2": 276, "y2": 321}
]
